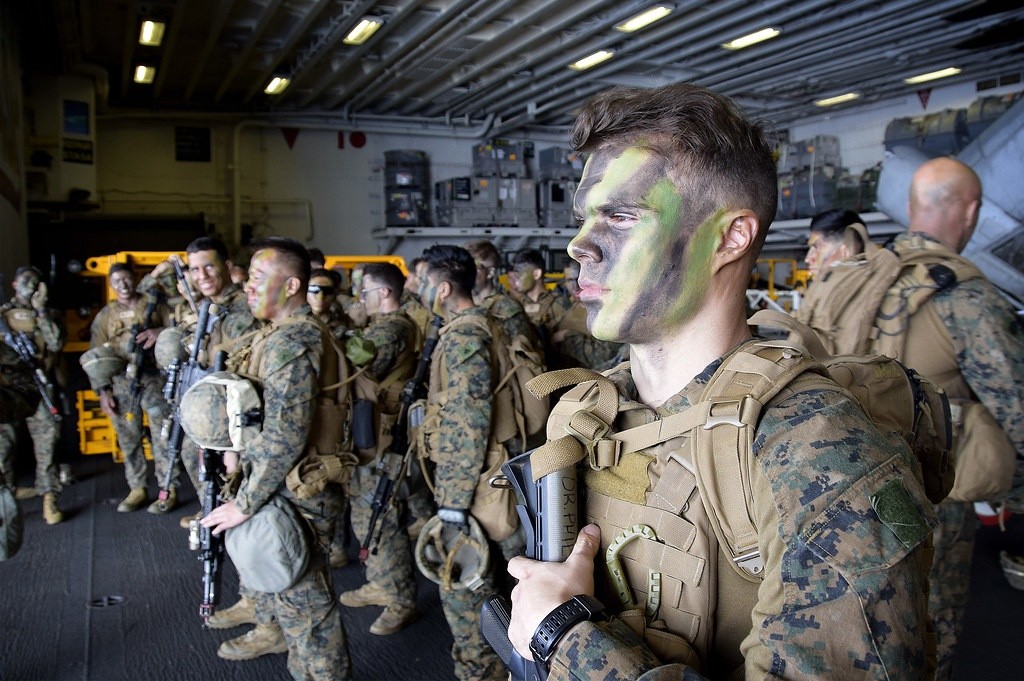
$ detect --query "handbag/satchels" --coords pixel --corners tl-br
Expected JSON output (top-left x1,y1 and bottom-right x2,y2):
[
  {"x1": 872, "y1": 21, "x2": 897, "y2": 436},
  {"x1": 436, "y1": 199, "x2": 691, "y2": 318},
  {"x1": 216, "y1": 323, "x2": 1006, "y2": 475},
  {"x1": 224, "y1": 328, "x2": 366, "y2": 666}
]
[
  {"x1": 946, "y1": 405, "x2": 1015, "y2": 501},
  {"x1": 223, "y1": 494, "x2": 309, "y2": 593},
  {"x1": 469, "y1": 442, "x2": 520, "y2": 541},
  {"x1": 0, "y1": 484, "x2": 24, "y2": 561}
]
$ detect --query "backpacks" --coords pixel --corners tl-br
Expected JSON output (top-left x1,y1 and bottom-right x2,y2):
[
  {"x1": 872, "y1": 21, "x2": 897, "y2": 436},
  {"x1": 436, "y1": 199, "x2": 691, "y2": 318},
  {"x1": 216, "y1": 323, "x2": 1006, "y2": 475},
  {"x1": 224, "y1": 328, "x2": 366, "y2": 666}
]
[
  {"x1": 691, "y1": 310, "x2": 954, "y2": 681},
  {"x1": 788, "y1": 248, "x2": 976, "y2": 363},
  {"x1": 439, "y1": 316, "x2": 550, "y2": 436}
]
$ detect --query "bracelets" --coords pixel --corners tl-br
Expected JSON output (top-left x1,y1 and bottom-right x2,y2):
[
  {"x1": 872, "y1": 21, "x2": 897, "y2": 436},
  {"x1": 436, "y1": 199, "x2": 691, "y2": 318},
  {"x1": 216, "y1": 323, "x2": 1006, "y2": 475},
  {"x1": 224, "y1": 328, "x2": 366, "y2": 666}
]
[{"x1": 527, "y1": 594, "x2": 611, "y2": 681}]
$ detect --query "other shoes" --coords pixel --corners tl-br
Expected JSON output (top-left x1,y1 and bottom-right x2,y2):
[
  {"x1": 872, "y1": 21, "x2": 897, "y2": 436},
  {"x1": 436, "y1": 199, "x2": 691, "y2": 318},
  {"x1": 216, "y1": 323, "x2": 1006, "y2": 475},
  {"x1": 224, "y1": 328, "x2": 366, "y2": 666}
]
[{"x1": 329, "y1": 548, "x2": 348, "y2": 567}]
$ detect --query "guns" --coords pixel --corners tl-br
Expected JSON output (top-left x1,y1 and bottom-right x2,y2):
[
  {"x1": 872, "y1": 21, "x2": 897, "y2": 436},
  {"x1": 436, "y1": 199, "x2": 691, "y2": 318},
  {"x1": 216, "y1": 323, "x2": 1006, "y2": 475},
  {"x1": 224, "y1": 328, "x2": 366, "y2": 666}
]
[
  {"x1": 479, "y1": 445, "x2": 580, "y2": 681},
  {"x1": 358, "y1": 316, "x2": 444, "y2": 565},
  {"x1": 0, "y1": 316, "x2": 63, "y2": 420},
  {"x1": 190, "y1": 350, "x2": 228, "y2": 621},
  {"x1": 158, "y1": 300, "x2": 226, "y2": 506},
  {"x1": 170, "y1": 256, "x2": 200, "y2": 317},
  {"x1": 125, "y1": 285, "x2": 164, "y2": 423}
]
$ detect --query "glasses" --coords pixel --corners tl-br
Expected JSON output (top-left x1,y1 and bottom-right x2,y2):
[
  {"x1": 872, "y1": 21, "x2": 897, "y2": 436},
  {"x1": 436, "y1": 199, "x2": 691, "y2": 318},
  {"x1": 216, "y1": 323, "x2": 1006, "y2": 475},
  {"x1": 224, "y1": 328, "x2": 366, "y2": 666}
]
[
  {"x1": 361, "y1": 286, "x2": 391, "y2": 300},
  {"x1": 308, "y1": 285, "x2": 337, "y2": 295}
]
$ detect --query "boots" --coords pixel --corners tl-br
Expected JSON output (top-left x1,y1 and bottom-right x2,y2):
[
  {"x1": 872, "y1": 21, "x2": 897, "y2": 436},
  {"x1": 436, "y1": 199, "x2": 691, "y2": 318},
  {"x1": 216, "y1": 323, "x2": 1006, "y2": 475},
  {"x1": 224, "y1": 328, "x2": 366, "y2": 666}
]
[
  {"x1": 217, "y1": 622, "x2": 289, "y2": 660},
  {"x1": 117, "y1": 487, "x2": 148, "y2": 512},
  {"x1": 339, "y1": 583, "x2": 388, "y2": 607},
  {"x1": 204, "y1": 597, "x2": 259, "y2": 629},
  {"x1": 148, "y1": 490, "x2": 178, "y2": 514},
  {"x1": 370, "y1": 597, "x2": 415, "y2": 635},
  {"x1": 42, "y1": 492, "x2": 62, "y2": 525},
  {"x1": 180, "y1": 511, "x2": 204, "y2": 528}
]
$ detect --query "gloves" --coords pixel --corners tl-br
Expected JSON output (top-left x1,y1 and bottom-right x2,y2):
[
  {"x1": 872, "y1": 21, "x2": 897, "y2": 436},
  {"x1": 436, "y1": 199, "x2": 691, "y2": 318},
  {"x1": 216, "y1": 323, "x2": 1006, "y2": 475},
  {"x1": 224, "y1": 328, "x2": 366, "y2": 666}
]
[{"x1": 439, "y1": 507, "x2": 470, "y2": 544}]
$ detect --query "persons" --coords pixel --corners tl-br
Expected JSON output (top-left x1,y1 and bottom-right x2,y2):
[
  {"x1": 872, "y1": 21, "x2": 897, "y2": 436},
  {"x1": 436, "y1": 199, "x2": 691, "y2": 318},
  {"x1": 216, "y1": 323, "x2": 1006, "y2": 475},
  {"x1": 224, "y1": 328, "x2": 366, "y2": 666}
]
[
  {"x1": 500, "y1": 84, "x2": 932, "y2": 681},
  {"x1": 0, "y1": 265, "x2": 66, "y2": 562},
  {"x1": 89, "y1": 262, "x2": 184, "y2": 513},
  {"x1": 174, "y1": 236, "x2": 624, "y2": 635},
  {"x1": 199, "y1": 238, "x2": 351, "y2": 681},
  {"x1": 805, "y1": 206, "x2": 882, "y2": 300},
  {"x1": 790, "y1": 158, "x2": 1024, "y2": 681}
]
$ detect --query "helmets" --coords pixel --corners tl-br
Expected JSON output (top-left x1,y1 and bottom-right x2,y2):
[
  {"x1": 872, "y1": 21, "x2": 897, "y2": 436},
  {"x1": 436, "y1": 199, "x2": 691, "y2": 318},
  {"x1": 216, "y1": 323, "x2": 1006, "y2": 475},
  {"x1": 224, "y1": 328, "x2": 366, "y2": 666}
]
[
  {"x1": 80, "y1": 348, "x2": 123, "y2": 377},
  {"x1": 415, "y1": 511, "x2": 490, "y2": 588},
  {"x1": 155, "y1": 326, "x2": 188, "y2": 368},
  {"x1": 179, "y1": 371, "x2": 241, "y2": 447}
]
